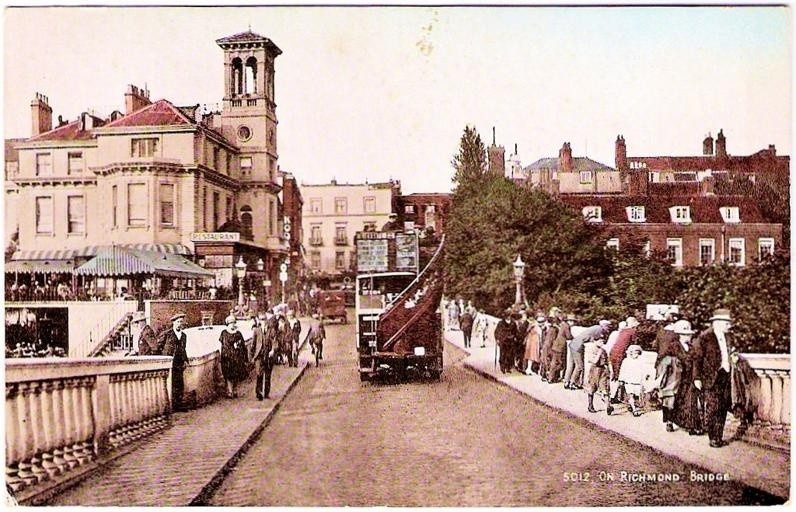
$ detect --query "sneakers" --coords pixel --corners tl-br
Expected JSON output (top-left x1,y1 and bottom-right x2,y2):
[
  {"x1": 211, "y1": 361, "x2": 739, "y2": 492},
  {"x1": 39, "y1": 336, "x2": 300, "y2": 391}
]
[
  {"x1": 606, "y1": 406, "x2": 615, "y2": 416},
  {"x1": 710, "y1": 439, "x2": 730, "y2": 448},
  {"x1": 664, "y1": 423, "x2": 675, "y2": 433},
  {"x1": 689, "y1": 427, "x2": 705, "y2": 437},
  {"x1": 226, "y1": 392, "x2": 271, "y2": 401},
  {"x1": 542, "y1": 376, "x2": 581, "y2": 392}
]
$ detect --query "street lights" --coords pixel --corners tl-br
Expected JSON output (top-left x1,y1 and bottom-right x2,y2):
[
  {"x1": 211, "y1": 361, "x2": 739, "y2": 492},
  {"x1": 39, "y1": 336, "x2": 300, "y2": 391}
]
[
  {"x1": 255, "y1": 257, "x2": 267, "y2": 320},
  {"x1": 233, "y1": 253, "x2": 249, "y2": 316},
  {"x1": 512, "y1": 252, "x2": 526, "y2": 303}
]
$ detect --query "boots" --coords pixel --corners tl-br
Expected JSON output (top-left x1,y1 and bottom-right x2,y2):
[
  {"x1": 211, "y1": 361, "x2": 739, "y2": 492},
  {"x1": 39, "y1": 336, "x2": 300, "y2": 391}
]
[{"x1": 587, "y1": 393, "x2": 597, "y2": 413}]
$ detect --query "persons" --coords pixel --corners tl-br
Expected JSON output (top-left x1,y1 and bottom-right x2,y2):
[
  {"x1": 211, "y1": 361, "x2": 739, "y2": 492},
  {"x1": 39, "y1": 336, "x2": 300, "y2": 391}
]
[
  {"x1": 215, "y1": 304, "x2": 325, "y2": 398},
  {"x1": 158, "y1": 312, "x2": 190, "y2": 406}
]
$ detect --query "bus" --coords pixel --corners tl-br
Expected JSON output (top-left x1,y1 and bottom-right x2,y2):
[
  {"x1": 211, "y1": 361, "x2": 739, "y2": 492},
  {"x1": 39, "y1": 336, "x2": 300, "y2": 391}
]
[
  {"x1": 352, "y1": 229, "x2": 444, "y2": 382},
  {"x1": 333, "y1": 284, "x2": 354, "y2": 306}
]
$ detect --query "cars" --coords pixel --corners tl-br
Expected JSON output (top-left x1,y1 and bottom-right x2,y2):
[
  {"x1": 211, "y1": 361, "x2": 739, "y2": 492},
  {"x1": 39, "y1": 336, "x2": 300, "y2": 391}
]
[{"x1": 314, "y1": 291, "x2": 346, "y2": 323}]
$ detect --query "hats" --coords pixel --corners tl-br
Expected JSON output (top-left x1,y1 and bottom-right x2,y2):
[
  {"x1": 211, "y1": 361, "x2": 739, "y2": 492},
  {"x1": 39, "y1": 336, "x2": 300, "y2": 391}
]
[
  {"x1": 673, "y1": 319, "x2": 697, "y2": 336},
  {"x1": 623, "y1": 317, "x2": 641, "y2": 329},
  {"x1": 258, "y1": 314, "x2": 267, "y2": 320},
  {"x1": 709, "y1": 308, "x2": 736, "y2": 322},
  {"x1": 170, "y1": 313, "x2": 186, "y2": 321},
  {"x1": 224, "y1": 314, "x2": 237, "y2": 324}
]
